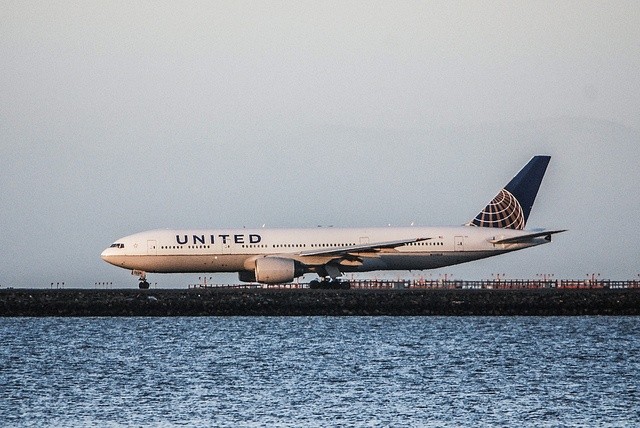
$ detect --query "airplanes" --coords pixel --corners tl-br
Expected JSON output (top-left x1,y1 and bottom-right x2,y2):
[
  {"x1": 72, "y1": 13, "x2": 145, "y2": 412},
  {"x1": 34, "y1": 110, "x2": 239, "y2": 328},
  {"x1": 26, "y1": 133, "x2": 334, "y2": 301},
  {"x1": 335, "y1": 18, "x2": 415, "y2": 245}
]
[{"x1": 100, "y1": 155, "x2": 569, "y2": 289}]
